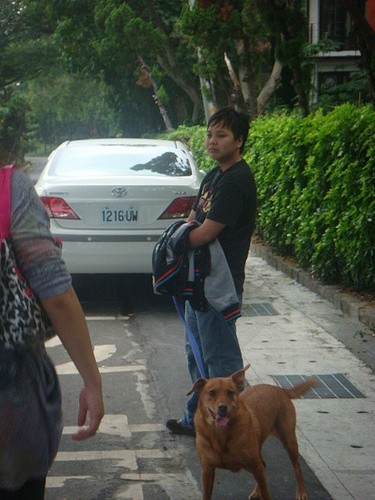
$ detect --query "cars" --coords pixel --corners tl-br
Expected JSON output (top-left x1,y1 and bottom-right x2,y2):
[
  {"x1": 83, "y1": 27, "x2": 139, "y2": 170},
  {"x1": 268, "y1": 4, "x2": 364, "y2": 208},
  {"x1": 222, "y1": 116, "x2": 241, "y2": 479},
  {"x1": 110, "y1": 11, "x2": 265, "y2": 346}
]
[{"x1": 33, "y1": 137, "x2": 206, "y2": 277}]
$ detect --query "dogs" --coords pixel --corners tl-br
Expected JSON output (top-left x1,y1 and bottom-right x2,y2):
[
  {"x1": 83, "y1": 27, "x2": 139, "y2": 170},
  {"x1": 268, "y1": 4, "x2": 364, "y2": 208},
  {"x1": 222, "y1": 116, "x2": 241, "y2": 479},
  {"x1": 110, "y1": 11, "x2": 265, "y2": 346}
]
[{"x1": 186, "y1": 363, "x2": 320, "y2": 500}]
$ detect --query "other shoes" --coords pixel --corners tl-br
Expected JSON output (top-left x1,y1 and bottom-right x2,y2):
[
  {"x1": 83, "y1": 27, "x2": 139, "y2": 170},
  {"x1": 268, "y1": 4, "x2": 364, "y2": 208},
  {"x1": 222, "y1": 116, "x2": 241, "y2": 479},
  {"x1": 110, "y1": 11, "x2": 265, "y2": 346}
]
[{"x1": 166, "y1": 418, "x2": 195, "y2": 436}]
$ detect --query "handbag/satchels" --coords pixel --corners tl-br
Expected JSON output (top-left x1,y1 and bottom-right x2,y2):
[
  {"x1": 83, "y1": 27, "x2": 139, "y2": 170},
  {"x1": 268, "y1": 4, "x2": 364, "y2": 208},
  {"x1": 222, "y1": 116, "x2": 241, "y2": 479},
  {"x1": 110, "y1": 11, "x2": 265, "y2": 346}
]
[{"x1": 1, "y1": 162, "x2": 57, "y2": 350}]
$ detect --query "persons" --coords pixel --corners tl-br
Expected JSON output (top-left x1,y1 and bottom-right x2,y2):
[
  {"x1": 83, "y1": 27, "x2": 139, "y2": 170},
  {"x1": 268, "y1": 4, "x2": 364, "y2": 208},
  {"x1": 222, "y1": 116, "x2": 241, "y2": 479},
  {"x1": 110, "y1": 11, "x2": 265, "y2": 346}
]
[
  {"x1": 0, "y1": 162, "x2": 105, "y2": 500},
  {"x1": 167, "y1": 106, "x2": 259, "y2": 434}
]
